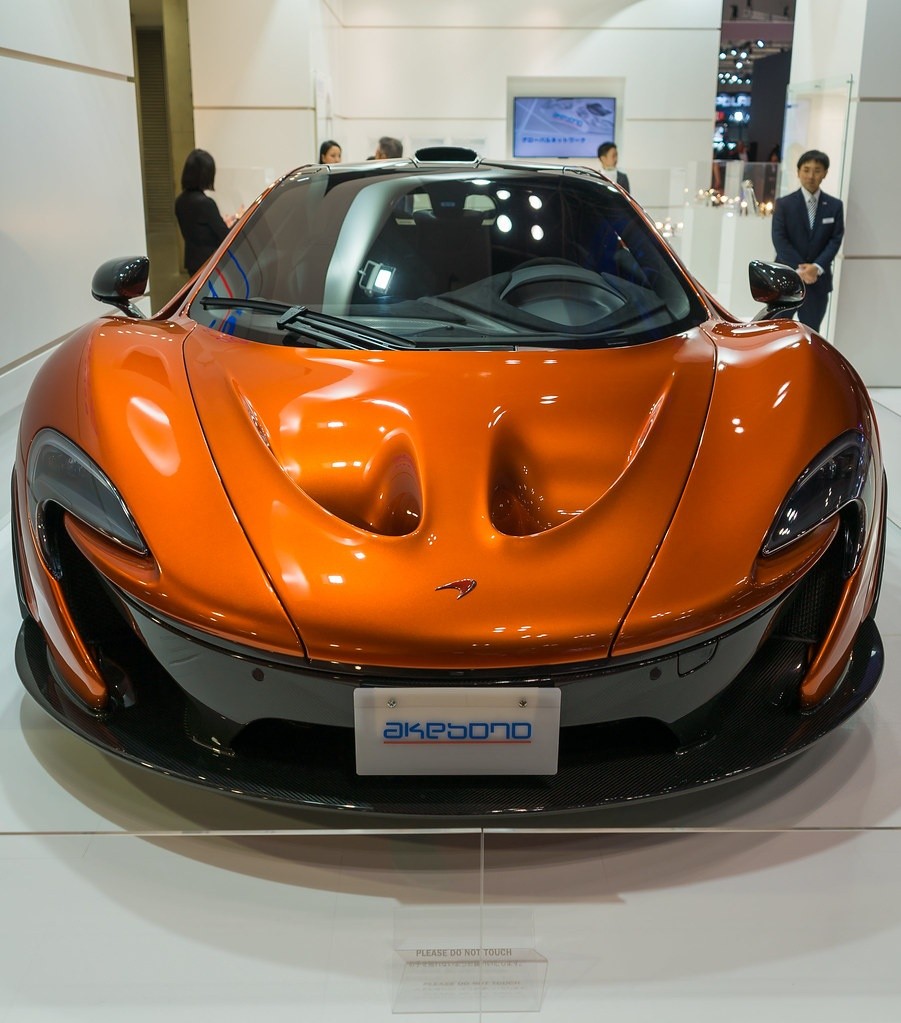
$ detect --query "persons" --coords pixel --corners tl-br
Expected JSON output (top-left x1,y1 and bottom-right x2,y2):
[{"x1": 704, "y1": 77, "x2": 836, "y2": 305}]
[
  {"x1": 375, "y1": 137, "x2": 413, "y2": 215},
  {"x1": 176, "y1": 149, "x2": 245, "y2": 276},
  {"x1": 597, "y1": 142, "x2": 629, "y2": 193},
  {"x1": 737, "y1": 144, "x2": 754, "y2": 162},
  {"x1": 772, "y1": 150, "x2": 844, "y2": 333},
  {"x1": 320, "y1": 140, "x2": 341, "y2": 164},
  {"x1": 768, "y1": 151, "x2": 779, "y2": 195}
]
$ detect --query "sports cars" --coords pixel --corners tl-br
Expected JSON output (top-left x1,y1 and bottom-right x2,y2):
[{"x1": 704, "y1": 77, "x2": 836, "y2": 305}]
[{"x1": 10, "y1": 143, "x2": 886, "y2": 814}]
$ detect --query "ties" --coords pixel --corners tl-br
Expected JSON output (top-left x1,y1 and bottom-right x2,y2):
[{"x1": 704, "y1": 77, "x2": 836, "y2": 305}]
[{"x1": 808, "y1": 196, "x2": 816, "y2": 230}]
